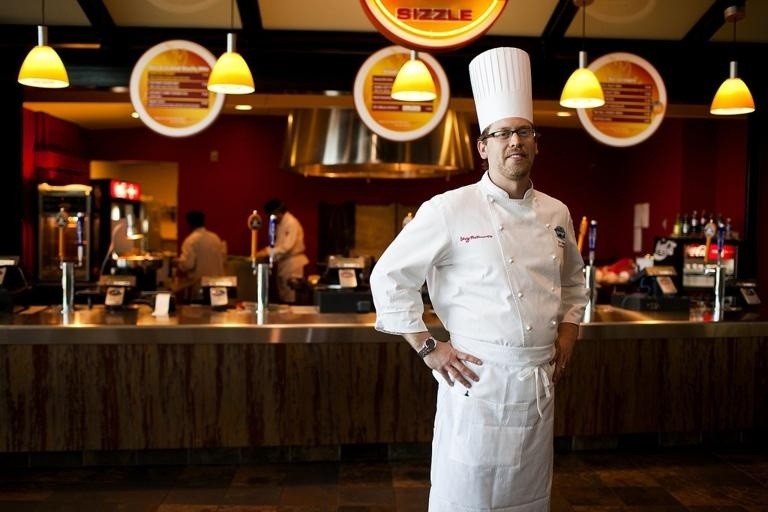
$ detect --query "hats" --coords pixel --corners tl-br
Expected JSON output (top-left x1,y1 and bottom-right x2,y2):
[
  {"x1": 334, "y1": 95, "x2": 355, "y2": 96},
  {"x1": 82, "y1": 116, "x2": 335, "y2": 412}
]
[{"x1": 469, "y1": 47, "x2": 533, "y2": 135}]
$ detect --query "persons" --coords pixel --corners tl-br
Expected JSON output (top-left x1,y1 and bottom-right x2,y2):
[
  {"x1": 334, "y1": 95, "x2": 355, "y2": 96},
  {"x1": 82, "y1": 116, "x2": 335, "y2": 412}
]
[
  {"x1": 100, "y1": 205, "x2": 153, "y2": 286},
  {"x1": 170, "y1": 208, "x2": 235, "y2": 306},
  {"x1": 362, "y1": 116, "x2": 590, "y2": 511},
  {"x1": 247, "y1": 197, "x2": 310, "y2": 301}
]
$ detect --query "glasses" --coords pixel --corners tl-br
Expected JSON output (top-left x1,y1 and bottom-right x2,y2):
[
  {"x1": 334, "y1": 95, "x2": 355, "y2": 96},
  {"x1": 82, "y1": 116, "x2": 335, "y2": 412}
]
[{"x1": 481, "y1": 127, "x2": 536, "y2": 141}]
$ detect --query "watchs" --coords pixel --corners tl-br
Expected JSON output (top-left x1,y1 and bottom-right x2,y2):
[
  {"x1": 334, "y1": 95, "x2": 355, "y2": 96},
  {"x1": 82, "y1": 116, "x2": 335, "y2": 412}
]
[{"x1": 412, "y1": 337, "x2": 438, "y2": 358}]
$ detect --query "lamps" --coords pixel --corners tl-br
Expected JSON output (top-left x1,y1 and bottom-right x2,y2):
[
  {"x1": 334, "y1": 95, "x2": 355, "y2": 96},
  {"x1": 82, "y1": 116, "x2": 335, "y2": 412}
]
[
  {"x1": 559, "y1": 0, "x2": 606, "y2": 110},
  {"x1": 390, "y1": 49, "x2": 438, "y2": 103},
  {"x1": 206, "y1": 0, "x2": 256, "y2": 95},
  {"x1": 709, "y1": 5, "x2": 756, "y2": 116},
  {"x1": 17, "y1": 1, "x2": 71, "y2": 88}
]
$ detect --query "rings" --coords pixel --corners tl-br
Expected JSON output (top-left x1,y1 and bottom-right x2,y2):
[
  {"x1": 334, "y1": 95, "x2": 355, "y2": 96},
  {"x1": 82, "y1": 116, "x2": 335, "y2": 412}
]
[{"x1": 561, "y1": 363, "x2": 566, "y2": 369}]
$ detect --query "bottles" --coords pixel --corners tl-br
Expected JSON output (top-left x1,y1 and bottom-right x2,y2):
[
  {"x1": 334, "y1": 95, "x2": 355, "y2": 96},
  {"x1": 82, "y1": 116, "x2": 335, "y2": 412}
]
[{"x1": 672, "y1": 209, "x2": 734, "y2": 240}]
